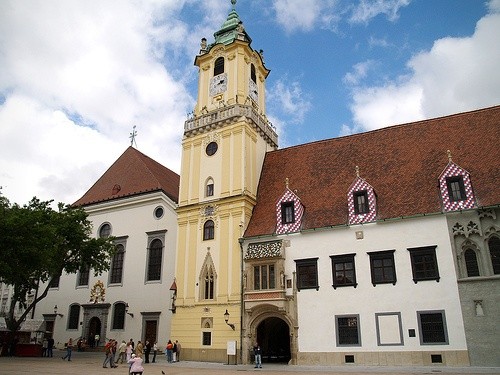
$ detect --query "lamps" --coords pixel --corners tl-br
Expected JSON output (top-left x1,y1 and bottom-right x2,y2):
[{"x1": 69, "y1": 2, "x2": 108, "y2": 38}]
[
  {"x1": 54, "y1": 304, "x2": 64, "y2": 318},
  {"x1": 224, "y1": 309, "x2": 235, "y2": 331},
  {"x1": 125, "y1": 302, "x2": 134, "y2": 318}
]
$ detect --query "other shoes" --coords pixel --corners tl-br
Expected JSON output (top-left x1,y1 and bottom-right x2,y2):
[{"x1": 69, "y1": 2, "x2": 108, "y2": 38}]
[
  {"x1": 254, "y1": 366, "x2": 262, "y2": 368},
  {"x1": 103, "y1": 366, "x2": 118, "y2": 368}
]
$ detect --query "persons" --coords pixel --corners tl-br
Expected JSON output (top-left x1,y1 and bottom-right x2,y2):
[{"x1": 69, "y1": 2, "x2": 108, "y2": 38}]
[
  {"x1": 253, "y1": 342, "x2": 262, "y2": 368},
  {"x1": 63, "y1": 338, "x2": 73, "y2": 361},
  {"x1": 77, "y1": 332, "x2": 100, "y2": 351},
  {"x1": 2, "y1": 335, "x2": 55, "y2": 357},
  {"x1": 103, "y1": 339, "x2": 182, "y2": 375}
]
